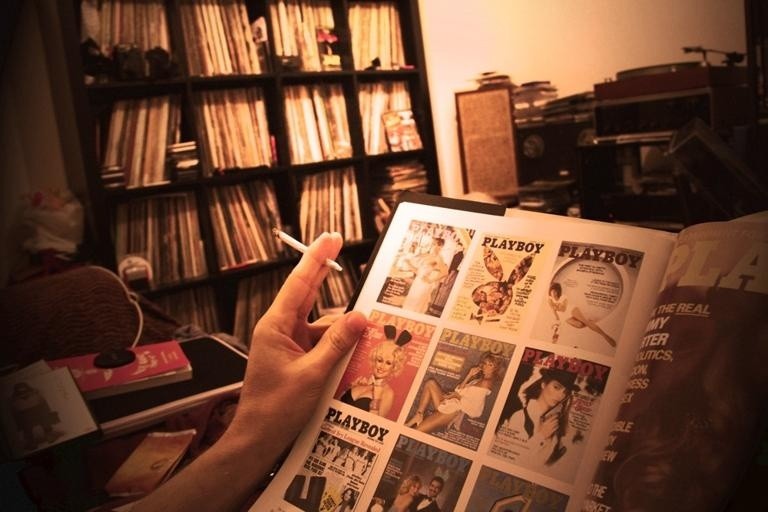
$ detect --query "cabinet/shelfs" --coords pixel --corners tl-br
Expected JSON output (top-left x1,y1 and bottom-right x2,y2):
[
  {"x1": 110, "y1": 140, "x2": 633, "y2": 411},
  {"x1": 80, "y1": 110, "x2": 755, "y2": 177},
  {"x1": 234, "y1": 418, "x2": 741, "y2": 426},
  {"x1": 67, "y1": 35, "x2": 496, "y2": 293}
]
[
  {"x1": 594, "y1": 86, "x2": 753, "y2": 230},
  {"x1": 43, "y1": 0, "x2": 442, "y2": 354}
]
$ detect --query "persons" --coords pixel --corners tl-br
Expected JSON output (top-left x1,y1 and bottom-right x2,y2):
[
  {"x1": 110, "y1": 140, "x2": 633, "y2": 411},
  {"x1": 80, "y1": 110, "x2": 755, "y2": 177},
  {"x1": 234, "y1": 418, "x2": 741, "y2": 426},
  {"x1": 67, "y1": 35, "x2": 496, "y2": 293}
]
[
  {"x1": 547, "y1": 281, "x2": 619, "y2": 354},
  {"x1": 295, "y1": 322, "x2": 579, "y2": 512},
  {"x1": 100, "y1": 233, "x2": 367, "y2": 512},
  {"x1": 80, "y1": 2, "x2": 430, "y2": 354},
  {"x1": 396, "y1": 235, "x2": 449, "y2": 311}
]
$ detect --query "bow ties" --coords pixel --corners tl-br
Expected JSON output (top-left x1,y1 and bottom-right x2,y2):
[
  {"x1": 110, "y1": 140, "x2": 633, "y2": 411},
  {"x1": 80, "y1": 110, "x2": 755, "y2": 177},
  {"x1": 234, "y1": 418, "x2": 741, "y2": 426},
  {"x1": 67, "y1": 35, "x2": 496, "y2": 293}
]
[{"x1": 424, "y1": 494, "x2": 433, "y2": 502}]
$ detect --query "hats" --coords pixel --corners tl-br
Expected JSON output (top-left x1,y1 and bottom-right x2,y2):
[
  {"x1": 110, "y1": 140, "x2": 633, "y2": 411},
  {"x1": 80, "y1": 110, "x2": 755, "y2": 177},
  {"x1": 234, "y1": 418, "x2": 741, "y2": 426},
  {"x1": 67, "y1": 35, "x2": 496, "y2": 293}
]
[{"x1": 540, "y1": 360, "x2": 580, "y2": 392}]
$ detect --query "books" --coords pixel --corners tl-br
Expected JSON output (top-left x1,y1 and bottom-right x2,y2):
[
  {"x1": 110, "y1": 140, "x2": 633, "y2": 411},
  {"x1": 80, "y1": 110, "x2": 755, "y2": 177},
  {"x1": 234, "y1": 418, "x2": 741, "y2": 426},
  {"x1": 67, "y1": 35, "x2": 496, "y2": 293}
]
[
  {"x1": 1, "y1": 367, "x2": 99, "y2": 463},
  {"x1": 479, "y1": 71, "x2": 677, "y2": 216},
  {"x1": 46, "y1": 340, "x2": 193, "y2": 400},
  {"x1": 0, "y1": 360, "x2": 55, "y2": 381},
  {"x1": 238, "y1": 188, "x2": 768, "y2": 512}
]
